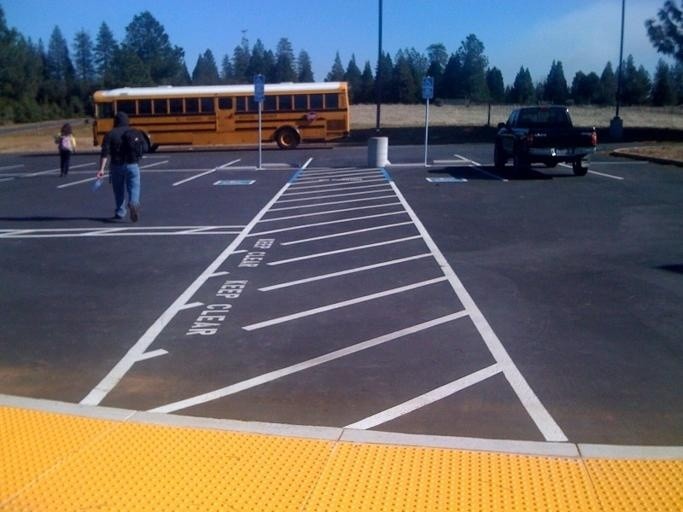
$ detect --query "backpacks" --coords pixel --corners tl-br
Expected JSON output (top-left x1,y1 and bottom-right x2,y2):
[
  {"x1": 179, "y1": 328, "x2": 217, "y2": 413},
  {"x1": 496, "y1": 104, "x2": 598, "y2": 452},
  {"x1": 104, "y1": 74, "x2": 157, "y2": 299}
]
[
  {"x1": 123, "y1": 129, "x2": 144, "y2": 162},
  {"x1": 59, "y1": 135, "x2": 72, "y2": 152}
]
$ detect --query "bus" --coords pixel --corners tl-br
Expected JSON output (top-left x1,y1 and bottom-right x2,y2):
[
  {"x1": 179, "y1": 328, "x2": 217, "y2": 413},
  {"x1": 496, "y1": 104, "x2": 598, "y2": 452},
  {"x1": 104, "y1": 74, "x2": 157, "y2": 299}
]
[{"x1": 91, "y1": 81, "x2": 349, "y2": 150}]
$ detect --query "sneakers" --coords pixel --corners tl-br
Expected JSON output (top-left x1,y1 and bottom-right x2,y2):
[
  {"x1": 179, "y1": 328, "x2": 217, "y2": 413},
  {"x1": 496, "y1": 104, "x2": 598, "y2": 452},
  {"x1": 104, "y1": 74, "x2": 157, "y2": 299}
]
[{"x1": 108, "y1": 206, "x2": 139, "y2": 223}]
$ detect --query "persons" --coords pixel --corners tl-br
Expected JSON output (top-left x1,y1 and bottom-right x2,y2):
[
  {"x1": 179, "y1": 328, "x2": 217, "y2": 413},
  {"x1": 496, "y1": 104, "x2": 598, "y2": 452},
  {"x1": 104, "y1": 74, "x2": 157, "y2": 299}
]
[
  {"x1": 54, "y1": 122, "x2": 78, "y2": 178},
  {"x1": 95, "y1": 110, "x2": 139, "y2": 225}
]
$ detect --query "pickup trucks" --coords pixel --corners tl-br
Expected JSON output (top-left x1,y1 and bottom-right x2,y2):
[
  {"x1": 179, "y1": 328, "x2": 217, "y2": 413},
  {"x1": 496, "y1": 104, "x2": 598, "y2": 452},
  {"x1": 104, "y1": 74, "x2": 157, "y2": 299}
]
[{"x1": 494, "y1": 106, "x2": 597, "y2": 176}]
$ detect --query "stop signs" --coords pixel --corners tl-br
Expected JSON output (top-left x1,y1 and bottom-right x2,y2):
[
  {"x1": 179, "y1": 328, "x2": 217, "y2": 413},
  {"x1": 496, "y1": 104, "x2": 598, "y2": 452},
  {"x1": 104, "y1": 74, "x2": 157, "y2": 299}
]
[{"x1": 307, "y1": 112, "x2": 318, "y2": 123}]
